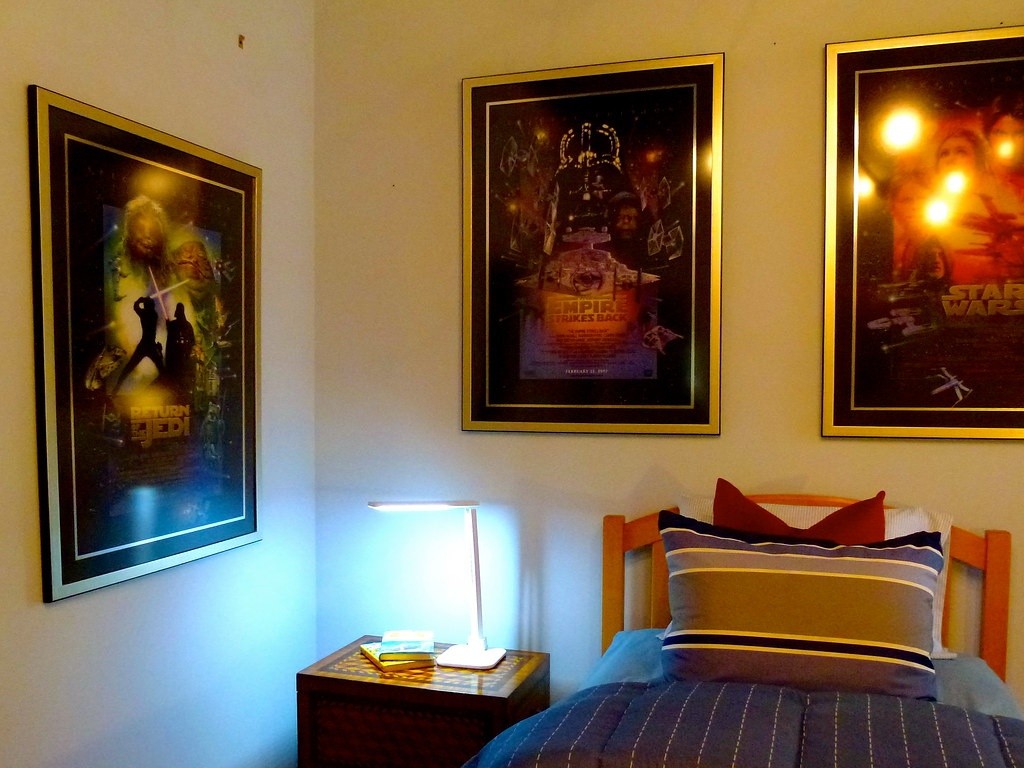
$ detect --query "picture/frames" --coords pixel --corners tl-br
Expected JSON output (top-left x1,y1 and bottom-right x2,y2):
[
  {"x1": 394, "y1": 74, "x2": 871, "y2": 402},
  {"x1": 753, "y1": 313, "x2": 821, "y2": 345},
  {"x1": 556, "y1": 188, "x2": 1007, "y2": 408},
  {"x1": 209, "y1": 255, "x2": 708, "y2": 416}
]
[
  {"x1": 821, "y1": 25, "x2": 1024, "y2": 439},
  {"x1": 27, "y1": 85, "x2": 263, "y2": 603},
  {"x1": 461, "y1": 52, "x2": 726, "y2": 436}
]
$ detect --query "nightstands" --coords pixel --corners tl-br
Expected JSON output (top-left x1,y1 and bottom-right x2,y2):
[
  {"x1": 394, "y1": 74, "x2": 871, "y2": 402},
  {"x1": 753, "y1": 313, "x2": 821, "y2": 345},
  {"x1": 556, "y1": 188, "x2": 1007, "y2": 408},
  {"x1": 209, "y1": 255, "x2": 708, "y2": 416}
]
[{"x1": 296, "y1": 635, "x2": 550, "y2": 768}]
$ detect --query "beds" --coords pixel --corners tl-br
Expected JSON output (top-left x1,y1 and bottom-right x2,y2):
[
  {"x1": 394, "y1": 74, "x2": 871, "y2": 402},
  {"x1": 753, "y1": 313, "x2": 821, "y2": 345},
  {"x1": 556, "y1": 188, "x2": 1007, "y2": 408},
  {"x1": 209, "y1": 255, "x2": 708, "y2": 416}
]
[{"x1": 462, "y1": 494, "x2": 1024, "y2": 768}]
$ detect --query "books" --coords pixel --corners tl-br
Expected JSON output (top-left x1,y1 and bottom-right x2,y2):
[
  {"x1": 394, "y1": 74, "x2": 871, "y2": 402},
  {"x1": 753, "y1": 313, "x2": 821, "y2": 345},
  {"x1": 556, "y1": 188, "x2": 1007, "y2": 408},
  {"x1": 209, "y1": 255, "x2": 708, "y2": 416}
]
[
  {"x1": 380, "y1": 629, "x2": 435, "y2": 660},
  {"x1": 360, "y1": 642, "x2": 436, "y2": 673}
]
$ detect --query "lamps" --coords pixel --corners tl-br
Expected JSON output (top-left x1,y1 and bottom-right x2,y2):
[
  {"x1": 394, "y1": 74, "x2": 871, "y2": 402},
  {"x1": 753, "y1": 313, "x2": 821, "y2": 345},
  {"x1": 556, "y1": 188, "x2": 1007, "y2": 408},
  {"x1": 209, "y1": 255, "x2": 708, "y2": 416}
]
[{"x1": 368, "y1": 500, "x2": 507, "y2": 669}]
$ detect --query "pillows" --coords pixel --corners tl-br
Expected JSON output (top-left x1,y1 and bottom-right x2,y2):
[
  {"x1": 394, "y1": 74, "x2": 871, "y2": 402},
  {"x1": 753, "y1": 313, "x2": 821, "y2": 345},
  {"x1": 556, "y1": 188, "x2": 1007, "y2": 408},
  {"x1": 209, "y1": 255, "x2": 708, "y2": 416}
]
[{"x1": 658, "y1": 477, "x2": 954, "y2": 701}]
